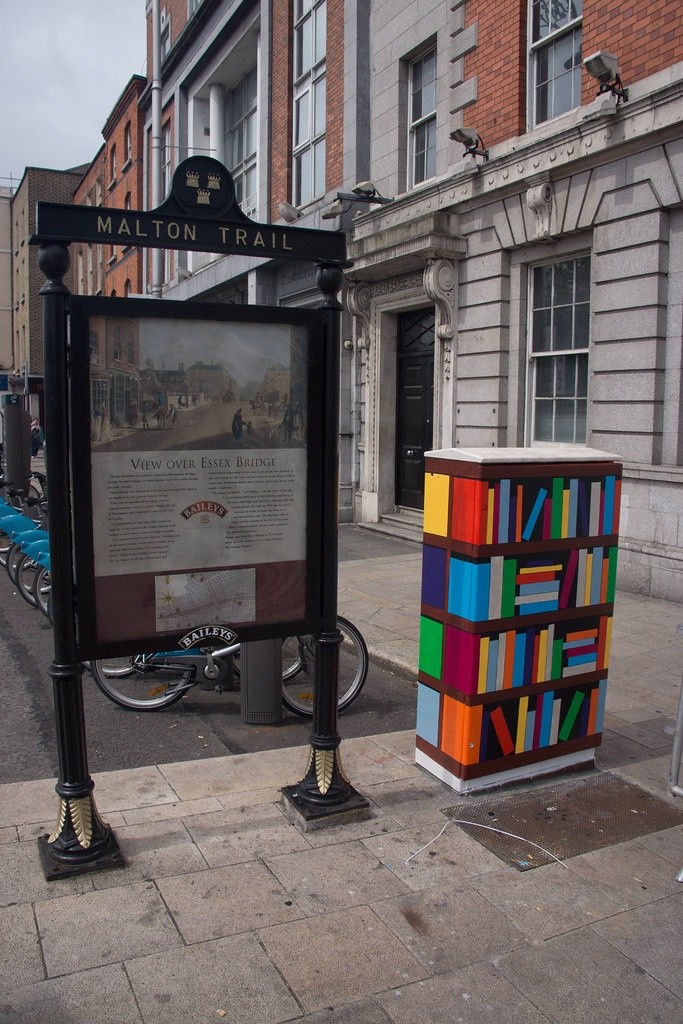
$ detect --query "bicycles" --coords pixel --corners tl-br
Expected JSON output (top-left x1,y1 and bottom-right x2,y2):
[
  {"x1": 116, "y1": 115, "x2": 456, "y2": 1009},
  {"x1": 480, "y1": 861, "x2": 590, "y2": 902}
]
[{"x1": 0, "y1": 472, "x2": 369, "y2": 716}]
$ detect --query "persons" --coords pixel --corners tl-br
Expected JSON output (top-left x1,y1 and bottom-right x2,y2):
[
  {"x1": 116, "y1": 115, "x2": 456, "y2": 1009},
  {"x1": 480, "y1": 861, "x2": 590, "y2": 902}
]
[{"x1": 31, "y1": 417, "x2": 41, "y2": 459}]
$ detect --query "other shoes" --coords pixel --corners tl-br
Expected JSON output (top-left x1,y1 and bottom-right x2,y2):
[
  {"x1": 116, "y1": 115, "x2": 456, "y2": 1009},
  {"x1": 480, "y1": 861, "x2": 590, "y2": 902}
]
[{"x1": 32, "y1": 456, "x2": 35, "y2": 458}]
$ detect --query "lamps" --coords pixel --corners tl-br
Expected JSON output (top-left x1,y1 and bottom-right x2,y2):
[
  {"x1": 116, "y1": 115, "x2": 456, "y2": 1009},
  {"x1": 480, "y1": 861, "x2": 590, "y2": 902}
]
[
  {"x1": 276, "y1": 201, "x2": 304, "y2": 224},
  {"x1": 583, "y1": 50, "x2": 629, "y2": 107},
  {"x1": 449, "y1": 127, "x2": 489, "y2": 162},
  {"x1": 175, "y1": 267, "x2": 193, "y2": 281},
  {"x1": 351, "y1": 182, "x2": 388, "y2": 206},
  {"x1": 322, "y1": 197, "x2": 359, "y2": 220}
]
